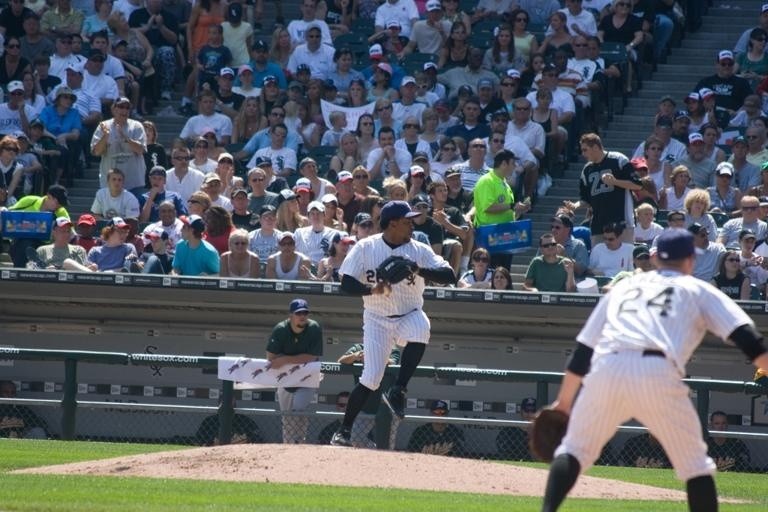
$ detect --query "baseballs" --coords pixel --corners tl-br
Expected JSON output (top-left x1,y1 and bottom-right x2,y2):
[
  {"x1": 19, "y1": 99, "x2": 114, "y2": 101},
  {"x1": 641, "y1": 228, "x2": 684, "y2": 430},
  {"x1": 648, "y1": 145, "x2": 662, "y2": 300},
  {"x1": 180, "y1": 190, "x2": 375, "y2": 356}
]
[{"x1": 383, "y1": 286, "x2": 392, "y2": 296}]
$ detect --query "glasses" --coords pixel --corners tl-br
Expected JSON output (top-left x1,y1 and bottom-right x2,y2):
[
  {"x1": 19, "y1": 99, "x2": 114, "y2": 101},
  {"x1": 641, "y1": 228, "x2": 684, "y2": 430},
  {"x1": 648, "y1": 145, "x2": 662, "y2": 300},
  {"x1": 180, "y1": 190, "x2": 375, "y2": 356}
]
[
  {"x1": 9, "y1": 44, "x2": 21, "y2": 50},
  {"x1": 14, "y1": 0, "x2": 25, "y2": 4},
  {"x1": 8, "y1": 90, "x2": 23, "y2": 96},
  {"x1": 337, "y1": 403, "x2": 346, "y2": 408},
  {"x1": 3, "y1": 147, "x2": 18, "y2": 152}
]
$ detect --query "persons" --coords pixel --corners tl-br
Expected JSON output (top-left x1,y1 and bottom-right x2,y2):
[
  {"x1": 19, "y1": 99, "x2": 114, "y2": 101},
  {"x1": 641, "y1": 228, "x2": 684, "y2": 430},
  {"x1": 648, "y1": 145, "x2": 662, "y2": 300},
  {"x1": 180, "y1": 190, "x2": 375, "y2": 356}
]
[
  {"x1": 318, "y1": 391, "x2": 373, "y2": 445},
  {"x1": 195, "y1": 394, "x2": 259, "y2": 446},
  {"x1": 495, "y1": 398, "x2": 537, "y2": 461},
  {"x1": 1, "y1": 0, "x2": 714, "y2": 289},
  {"x1": 617, "y1": 432, "x2": 672, "y2": 469},
  {"x1": 706, "y1": 411, "x2": 751, "y2": 472},
  {"x1": 409, "y1": 400, "x2": 464, "y2": 458},
  {"x1": 693, "y1": 2, "x2": 768, "y2": 301},
  {"x1": 338, "y1": 343, "x2": 399, "y2": 450},
  {"x1": 265, "y1": 298, "x2": 323, "y2": 444},
  {"x1": 542, "y1": 227, "x2": 768, "y2": 512},
  {"x1": 0, "y1": 380, "x2": 47, "y2": 439},
  {"x1": 330, "y1": 200, "x2": 457, "y2": 447}
]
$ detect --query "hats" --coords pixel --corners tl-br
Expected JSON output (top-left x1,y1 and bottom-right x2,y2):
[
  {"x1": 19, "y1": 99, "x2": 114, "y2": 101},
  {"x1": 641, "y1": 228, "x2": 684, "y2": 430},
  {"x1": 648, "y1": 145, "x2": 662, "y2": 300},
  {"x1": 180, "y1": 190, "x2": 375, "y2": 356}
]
[
  {"x1": 431, "y1": 400, "x2": 449, "y2": 409},
  {"x1": 289, "y1": 298, "x2": 310, "y2": 314},
  {"x1": 48, "y1": 183, "x2": 72, "y2": 207},
  {"x1": 51, "y1": 1, "x2": 767, "y2": 264},
  {"x1": 12, "y1": 130, "x2": 27, "y2": 140},
  {"x1": 6, "y1": 80, "x2": 25, "y2": 92},
  {"x1": 29, "y1": 118, "x2": 45, "y2": 127},
  {"x1": 24, "y1": 11, "x2": 38, "y2": 17},
  {"x1": 521, "y1": 397, "x2": 536, "y2": 410}
]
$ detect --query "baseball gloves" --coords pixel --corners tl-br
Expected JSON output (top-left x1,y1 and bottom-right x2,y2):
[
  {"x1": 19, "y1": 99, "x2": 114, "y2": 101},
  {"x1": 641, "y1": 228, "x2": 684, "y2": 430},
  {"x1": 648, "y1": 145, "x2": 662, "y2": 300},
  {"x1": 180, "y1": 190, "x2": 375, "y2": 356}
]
[
  {"x1": 376, "y1": 256, "x2": 417, "y2": 283},
  {"x1": 530, "y1": 409, "x2": 569, "y2": 464}
]
